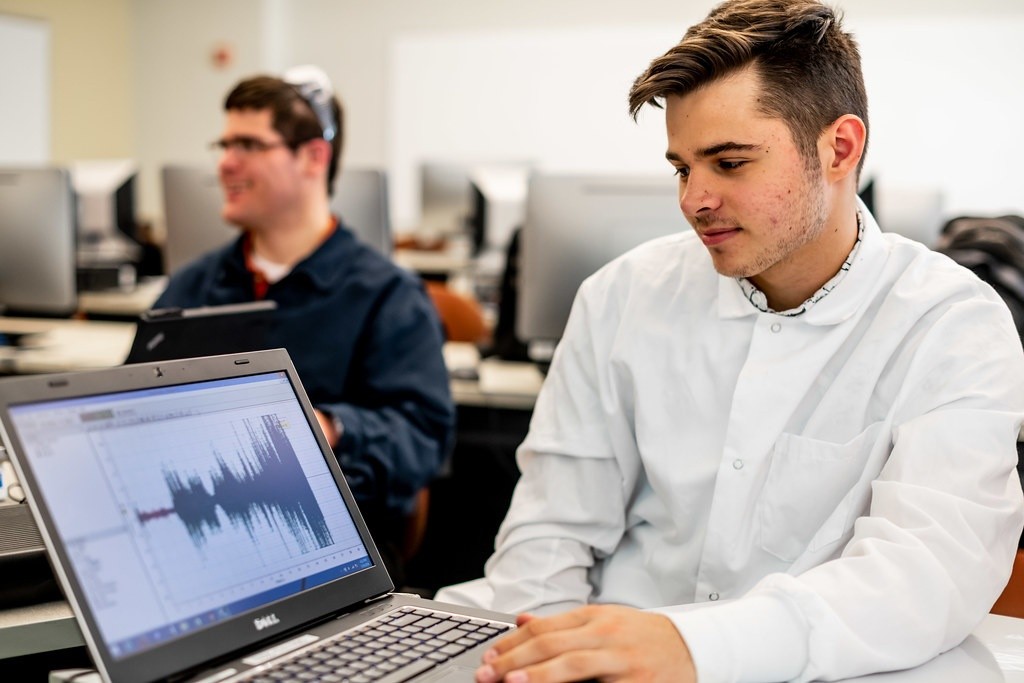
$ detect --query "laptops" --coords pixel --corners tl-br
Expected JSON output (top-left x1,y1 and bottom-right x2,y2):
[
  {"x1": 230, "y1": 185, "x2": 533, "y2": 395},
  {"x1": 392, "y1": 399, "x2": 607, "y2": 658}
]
[
  {"x1": 0, "y1": 159, "x2": 474, "y2": 315},
  {"x1": 516, "y1": 172, "x2": 694, "y2": 349},
  {"x1": 0, "y1": 348, "x2": 523, "y2": 683}
]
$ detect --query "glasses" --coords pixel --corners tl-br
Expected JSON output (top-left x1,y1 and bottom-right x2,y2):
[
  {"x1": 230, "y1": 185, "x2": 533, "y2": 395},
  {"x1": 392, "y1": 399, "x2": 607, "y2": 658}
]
[
  {"x1": 282, "y1": 64, "x2": 335, "y2": 132},
  {"x1": 212, "y1": 137, "x2": 287, "y2": 156}
]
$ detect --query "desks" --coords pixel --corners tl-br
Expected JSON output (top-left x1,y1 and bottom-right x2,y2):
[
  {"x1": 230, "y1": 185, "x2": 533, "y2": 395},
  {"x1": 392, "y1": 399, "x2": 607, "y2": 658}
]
[{"x1": 0, "y1": 250, "x2": 1024, "y2": 683}]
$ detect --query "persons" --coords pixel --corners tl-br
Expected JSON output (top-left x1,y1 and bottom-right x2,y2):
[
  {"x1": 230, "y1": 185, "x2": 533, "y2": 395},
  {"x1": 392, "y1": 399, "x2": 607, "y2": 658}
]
[
  {"x1": 115, "y1": 62, "x2": 457, "y2": 559},
  {"x1": 433, "y1": 1, "x2": 1024, "y2": 683}
]
